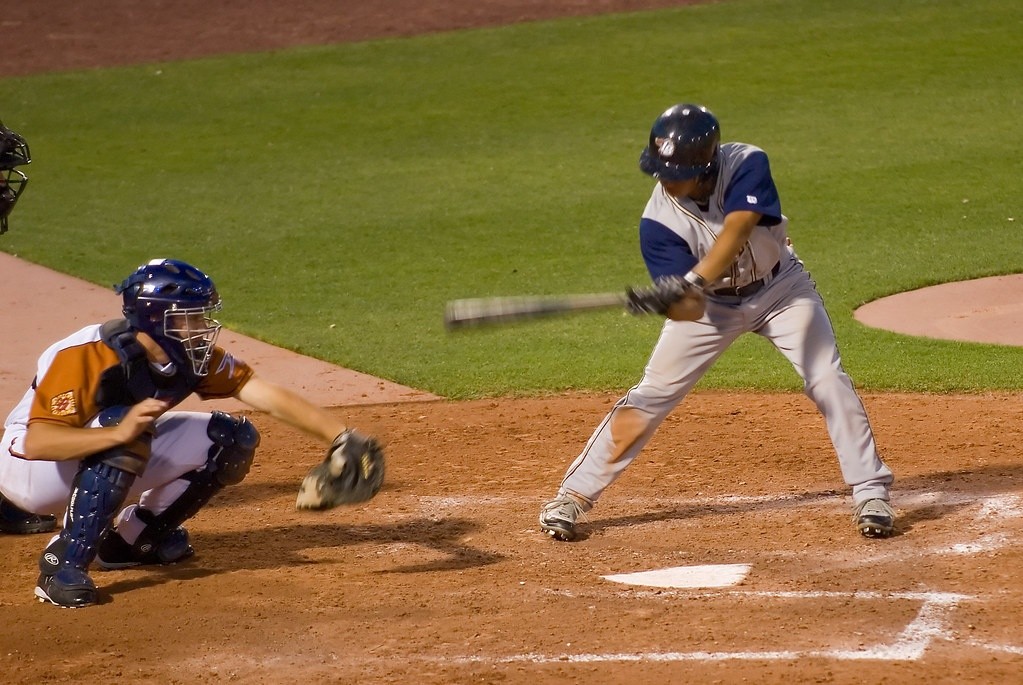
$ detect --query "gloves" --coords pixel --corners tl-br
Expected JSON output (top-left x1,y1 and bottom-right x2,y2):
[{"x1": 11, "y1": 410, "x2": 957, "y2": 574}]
[{"x1": 625, "y1": 271, "x2": 707, "y2": 319}]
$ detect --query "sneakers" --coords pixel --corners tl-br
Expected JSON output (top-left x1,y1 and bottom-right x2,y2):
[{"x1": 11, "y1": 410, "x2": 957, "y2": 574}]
[
  {"x1": 34, "y1": 572, "x2": 98, "y2": 609},
  {"x1": 539, "y1": 495, "x2": 591, "y2": 541},
  {"x1": 94, "y1": 526, "x2": 194, "y2": 569},
  {"x1": 851, "y1": 499, "x2": 899, "y2": 538},
  {"x1": 0, "y1": 490, "x2": 58, "y2": 535}
]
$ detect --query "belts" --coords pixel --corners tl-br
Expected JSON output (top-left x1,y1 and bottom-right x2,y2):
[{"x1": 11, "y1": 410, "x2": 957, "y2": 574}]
[{"x1": 714, "y1": 260, "x2": 781, "y2": 298}]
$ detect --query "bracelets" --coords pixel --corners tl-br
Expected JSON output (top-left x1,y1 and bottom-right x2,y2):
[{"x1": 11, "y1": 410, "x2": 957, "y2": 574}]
[{"x1": 682, "y1": 270, "x2": 707, "y2": 291}]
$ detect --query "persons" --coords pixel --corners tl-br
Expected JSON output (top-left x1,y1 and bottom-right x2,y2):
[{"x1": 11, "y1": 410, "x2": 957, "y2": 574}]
[
  {"x1": 540, "y1": 104, "x2": 896, "y2": 542},
  {"x1": 0, "y1": 119, "x2": 32, "y2": 235},
  {"x1": 0, "y1": 258, "x2": 386, "y2": 608}
]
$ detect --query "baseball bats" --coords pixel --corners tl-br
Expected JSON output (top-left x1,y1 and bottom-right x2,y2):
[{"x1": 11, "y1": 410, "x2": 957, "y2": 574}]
[{"x1": 444, "y1": 275, "x2": 743, "y2": 332}]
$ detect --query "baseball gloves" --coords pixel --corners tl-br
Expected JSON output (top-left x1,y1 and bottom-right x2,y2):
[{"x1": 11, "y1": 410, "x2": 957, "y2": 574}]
[{"x1": 295, "y1": 429, "x2": 385, "y2": 511}]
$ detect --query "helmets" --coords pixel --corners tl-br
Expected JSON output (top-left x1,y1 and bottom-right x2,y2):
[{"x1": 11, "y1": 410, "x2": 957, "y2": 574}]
[
  {"x1": 0, "y1": 122, "x2": 31, "y2": 236},
  {"x1": 117, "y1": 258, "x2": 220, "y2": 379},
  {"x1": 638, "y1": 104, "x2": 721, "y2": 181}
]
